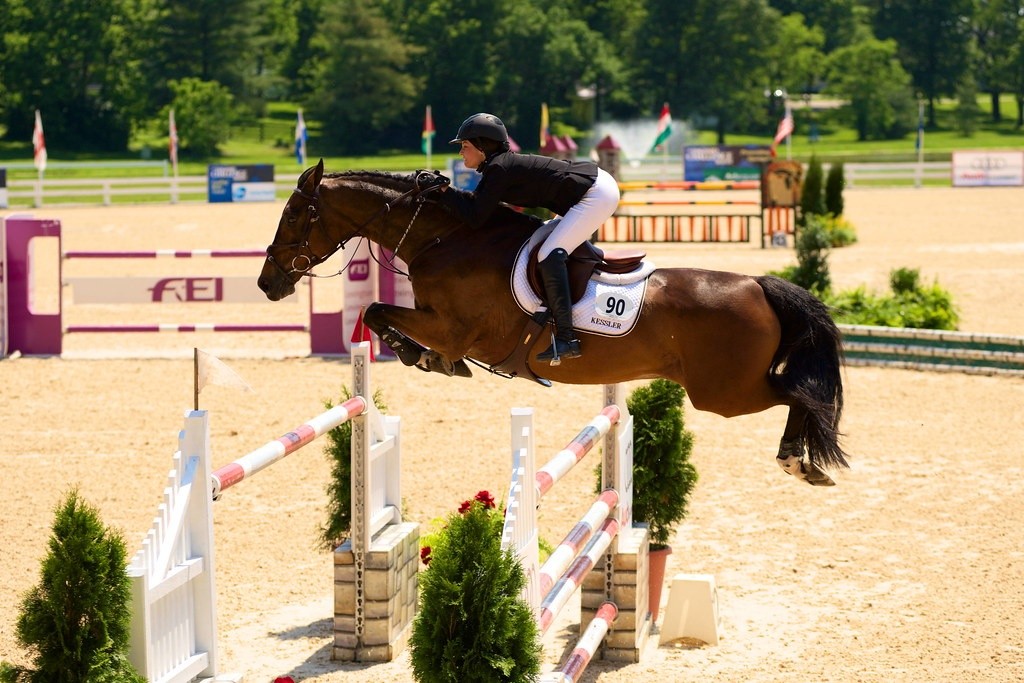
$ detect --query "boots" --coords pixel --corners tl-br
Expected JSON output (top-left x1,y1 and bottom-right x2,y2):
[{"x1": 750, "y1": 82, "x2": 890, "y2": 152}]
[{"x1": 536, "y1": 248, "x2": 582, "y2": 361}]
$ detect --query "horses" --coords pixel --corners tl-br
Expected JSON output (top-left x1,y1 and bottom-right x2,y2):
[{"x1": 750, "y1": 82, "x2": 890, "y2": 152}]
[{"x1": 255, "y1": 156, "x2": 853, "y2": 487}]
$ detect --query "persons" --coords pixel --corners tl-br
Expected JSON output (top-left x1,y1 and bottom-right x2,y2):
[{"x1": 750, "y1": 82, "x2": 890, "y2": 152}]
[{"x1": 432, "y1": 113, "x2": 620, "y2": 361}]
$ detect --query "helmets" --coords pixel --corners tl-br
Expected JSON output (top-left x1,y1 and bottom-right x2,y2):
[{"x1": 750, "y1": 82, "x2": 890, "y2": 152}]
[{"x1": 447, "y1": 113, "x2": 509, "y2": 156}]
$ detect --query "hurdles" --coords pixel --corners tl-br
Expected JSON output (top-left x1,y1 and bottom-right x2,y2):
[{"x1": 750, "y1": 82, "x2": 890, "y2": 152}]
[
  {"x1": 0, "y1": 213, "x2": 396, "y2": 361},
  {"x1": 587, "y1": 162, "x2": 806, "y2": 247},
  {"x1": 123, "y1": 339, "x2": 424, "y2": 683},
  {"x1": 499, "y1": 403, "x2": 652, "y2": 682}
]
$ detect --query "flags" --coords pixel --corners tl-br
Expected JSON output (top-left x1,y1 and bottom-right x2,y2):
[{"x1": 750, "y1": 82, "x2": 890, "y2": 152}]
[
  {"x1": 166, "y1": 110, "x2": 179, "y2": 160},
  {"x1": 539, "y1": 104, "x2": 550, "y2": 147},
  {"x1": 422, "y1": 108, "x2": 436, "y2": 155},
  {"x1": 652, "y1": 105, "x2": 674, "y2": 153},
  {"x1": 31, "y1": 111, "x2": 48, "y2": 169},
  {"x1": 766, "y1": 93, "x2": 793, "y2": 158},
  {"x1": 293, "y1": 114, "x2": 308, "y2": 165}
]
